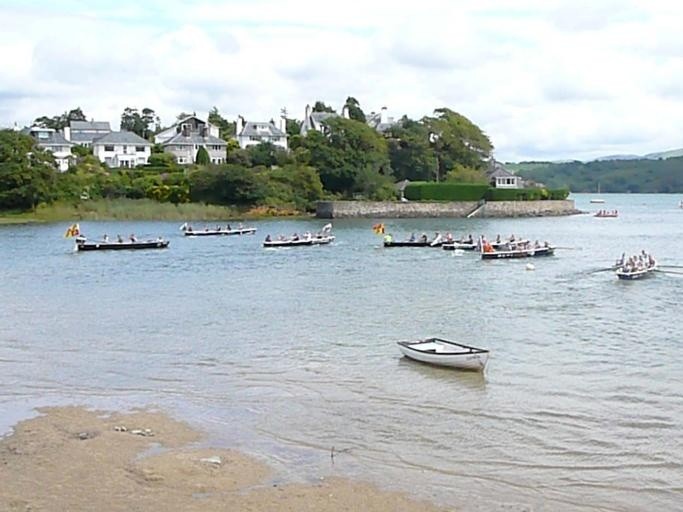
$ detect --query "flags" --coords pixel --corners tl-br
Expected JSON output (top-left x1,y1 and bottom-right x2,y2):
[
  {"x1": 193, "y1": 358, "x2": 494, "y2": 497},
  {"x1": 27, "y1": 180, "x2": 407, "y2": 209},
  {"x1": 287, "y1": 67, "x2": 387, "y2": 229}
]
[
  {"x1": 179, "y1": 222, "x2": 187, "y2": 230},
  {"x1": 64, "y1": 222, "x2": 79, "y2": 238},
  {"x1": 372, "y1": 223, "x2": 384, "y2": 234},
  {"x1": 323, "y1": 223, "x2": 332, "y2": 232}
]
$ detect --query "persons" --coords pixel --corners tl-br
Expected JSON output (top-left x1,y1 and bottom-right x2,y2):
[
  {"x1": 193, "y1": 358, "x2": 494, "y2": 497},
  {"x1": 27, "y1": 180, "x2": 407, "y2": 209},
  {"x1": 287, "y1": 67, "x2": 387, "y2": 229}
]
[
  {"x1": 408, "y1": 232, "x2": 416, "y2": 241},
  {"x1": 117, "y1": 233, "x2": 123, "y2": 244},
  {"x1": 265, "y1": 233, "x2": 271, "y2": 242},
  {"x1": 595, "y1": 208, "x2": 618, "y2": 216},
  {"x1": 291, "y1": 233, "x2": 298, "y2": 240},
  {"x1": 215, "y1": 224, "x2": 221, "y2": 231},
  {"x1": 203, "y1": 225, "x2": 208, "y2": 231},
  {"x1": 129, "y1": 234, "x2": 138, "y2": 242},
  {"x1": 420, "y1": 232, "x2": 548, "y2": 252},
  {"x1": 238, "y1": 222, "x2": 243, "y2": 230},
  {"x1": 103, "y1": 234, "x2": 111, "y2": 243},
  {"x1": 302, "y1": 231, "x2": 311, "y2": 240},
  {"x1": 384, "y1": 233, "x2": 392, "y2": 241},
  {"x1": 278, "y1": 234, "x2": 287, "y2": 242},
  {"x1": 622, "y1": 249, "x2": 656, "y2": 273},
  {"x1": 316, "y1": 232, "x2": 322, "y2": 239},
  {"x1": 188, "y1": 223, "x2": 193, "y2": 231},
  {"x1": 226, "y1": 224, "x2": 231, "y2": 230},
  {"x1": 155, "y1": 235, "x2": 163, "y2": 243}
]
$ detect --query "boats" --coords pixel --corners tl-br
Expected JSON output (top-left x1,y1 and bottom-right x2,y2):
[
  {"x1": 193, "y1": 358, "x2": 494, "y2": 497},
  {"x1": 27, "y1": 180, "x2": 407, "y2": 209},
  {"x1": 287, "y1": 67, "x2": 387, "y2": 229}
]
[
  {"x1": 383, "y1": 237, "x2": 556, "y2": 261},
  {"x1": 616, "y1": 255, "x2": 657, "y2": 280},
  {"x1": 74, "y1": 236, "x2": 170, "y2": 251},
  {"x1": 262, "y1": 234, "x2": 337, "y2": 246},
  {"x1": 396, "y1": 336, "x2": 491, "y2": 372},
  {"x1": 184, "y1": 226, "x2": 257, "y2": 236}
]
